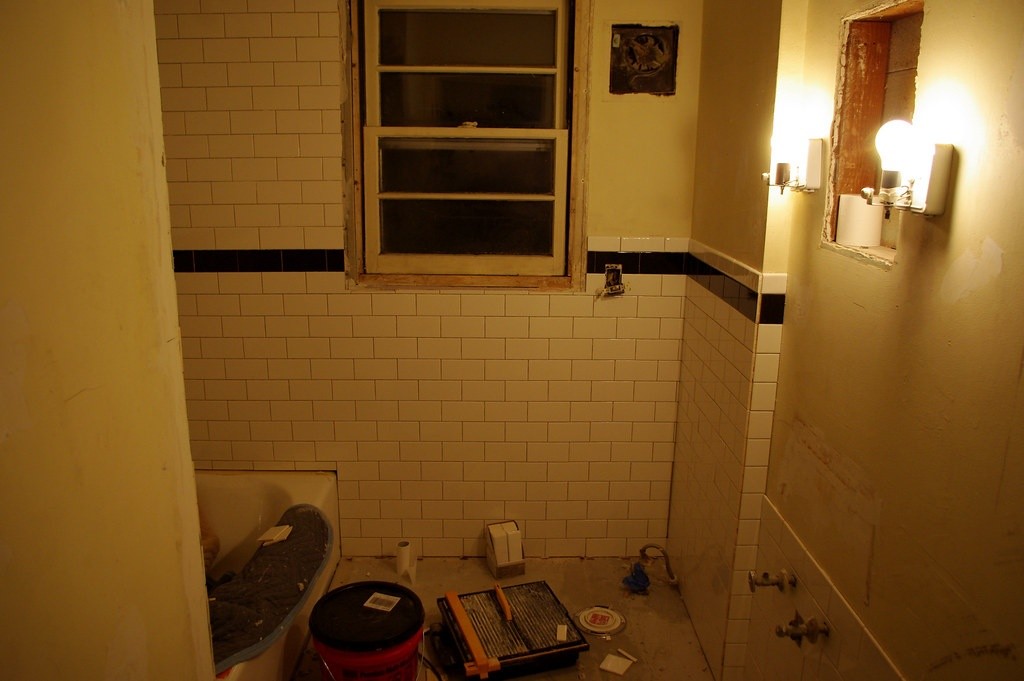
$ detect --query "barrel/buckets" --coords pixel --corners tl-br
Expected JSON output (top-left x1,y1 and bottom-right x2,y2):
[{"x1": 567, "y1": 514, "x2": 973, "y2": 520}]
[{"x1": 309, "y1": 580, "x2": 429, "y2": 680}]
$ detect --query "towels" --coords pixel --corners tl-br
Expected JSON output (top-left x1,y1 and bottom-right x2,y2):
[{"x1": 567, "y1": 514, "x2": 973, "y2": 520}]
[{"x1": 211, "y1": 504, "x2": 333, "y2": 672}]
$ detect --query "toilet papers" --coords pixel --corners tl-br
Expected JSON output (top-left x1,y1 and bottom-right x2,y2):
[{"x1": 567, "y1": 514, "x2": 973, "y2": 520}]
[
  {"x1": 837, "y1": 189, "x2": 885, "y2": 247},
  {"x1": 390, "y1": 540, "x2": 418, "y2": 582}
]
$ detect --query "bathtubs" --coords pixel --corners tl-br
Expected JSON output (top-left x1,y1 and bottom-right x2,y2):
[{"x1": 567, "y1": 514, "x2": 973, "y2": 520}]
[{"x1": 194, "y1": 469, "x2": 337, "y2": 680}]
